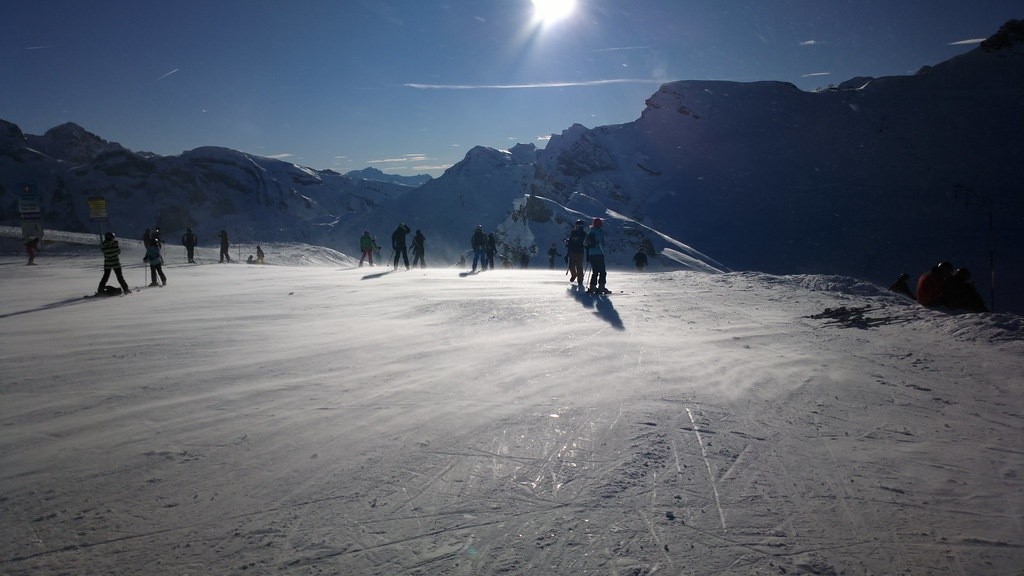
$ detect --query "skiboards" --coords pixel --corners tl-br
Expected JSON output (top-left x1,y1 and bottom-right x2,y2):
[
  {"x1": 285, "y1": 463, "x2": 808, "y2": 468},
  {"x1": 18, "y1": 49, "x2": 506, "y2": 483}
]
[
  {"x1": 391, "y1": 267, "x2": 411, "y2": 274},
  {"x1": 85, "y1": 289, "x2": 140, "y2": 300},
  {"x1": 587, "y1": 287, "x2": 632, "y2": 296},
  {"x1": 470, "y1": 268, "x2": 486, "y2": 274},
  {"x1": 186, "y1": 261, "x2": 197, "y2": 264},
  {"x1": 135, "y1": 284, "x2": 166, "y2": 289},
  {"x1": 358, "y1": 262, "x2": 374, "y2": 267}
]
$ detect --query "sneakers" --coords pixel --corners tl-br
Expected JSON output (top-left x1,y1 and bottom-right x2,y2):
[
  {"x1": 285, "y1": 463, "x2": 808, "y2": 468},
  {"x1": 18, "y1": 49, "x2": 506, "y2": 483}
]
[{"x1": 163, "y1": 280, "x2": 166, "y2": 285}]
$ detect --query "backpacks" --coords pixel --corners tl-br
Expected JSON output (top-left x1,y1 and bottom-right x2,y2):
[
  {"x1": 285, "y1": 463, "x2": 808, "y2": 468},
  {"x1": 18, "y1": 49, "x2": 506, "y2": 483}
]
[{"x1": 586, "y1": 231, "x2": 599, "y2": 248}]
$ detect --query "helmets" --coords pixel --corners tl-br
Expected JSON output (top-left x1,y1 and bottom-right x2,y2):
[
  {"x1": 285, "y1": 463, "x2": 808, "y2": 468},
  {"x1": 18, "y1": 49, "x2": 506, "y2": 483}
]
[
  {"x1": 575, "y1": 220, "x2": 585, "y2": 226},
  {"x1": 593, "y1": 218, "x2": 603, "y2": 227}
]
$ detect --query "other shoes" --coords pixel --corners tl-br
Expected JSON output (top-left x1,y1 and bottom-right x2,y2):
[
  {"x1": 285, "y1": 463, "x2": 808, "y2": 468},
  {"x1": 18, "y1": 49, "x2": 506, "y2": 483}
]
[
  {"x1": 570, "y1": 275, "x2": 576, "y2": 282},
  {"x1": 192, "y1": 260, "x2": 195, "y2": 263},
  {"x1": 590, "y1": 286, "x2": 611, "y2": 294}
]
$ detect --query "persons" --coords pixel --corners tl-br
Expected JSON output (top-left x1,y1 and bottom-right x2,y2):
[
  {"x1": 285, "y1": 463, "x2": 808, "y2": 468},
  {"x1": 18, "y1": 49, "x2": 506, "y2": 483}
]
[
  {"x1": 564, "y1": 218, "x2": 589, "y2": 286},
  {"x1": 520, "y1": 252, "x2": 529, "y2": 268},
  {"x1": 97, "y1": 232, "x2": 131, "y2": 296},
  {"x1": 256, "y1": 245, "x2": 265, "y2": 263},
  {"x1": 583, "y1": 218, "x2": 612, "y2": 295},
  {"x1": 547, "y1": 242, "x2": 562, "y2": 269},
  {"x1": 151, "y1": 226, "x2": 166, "y2": 249},
  {"x1": 891, "y1": 263, "x2": 989, "y2": 314},
  {"x1": 632, "y1": 246, "x2": 648, "y2": 272},
  {"x1": 246, "y1": 255, "x2": 257, "y2": 263},
  {"x1": 26, "y1": 238, "x2": 40, "y2": 265},
  {"x1": 217, "y1": 232, "x2": 230, "y2": 263},
  {"x1": 142, "y1": 238, "x2": 168, "y2": 287},
  {"x1": 143, "y1": 228, "x2": 150, "y2": 250},
  {"x1": 357, "y1": 221, "x2": 513, "y2": 270},
  {"x1": 182, "y1": 228, "x2": 197, "y2": 264}
]
